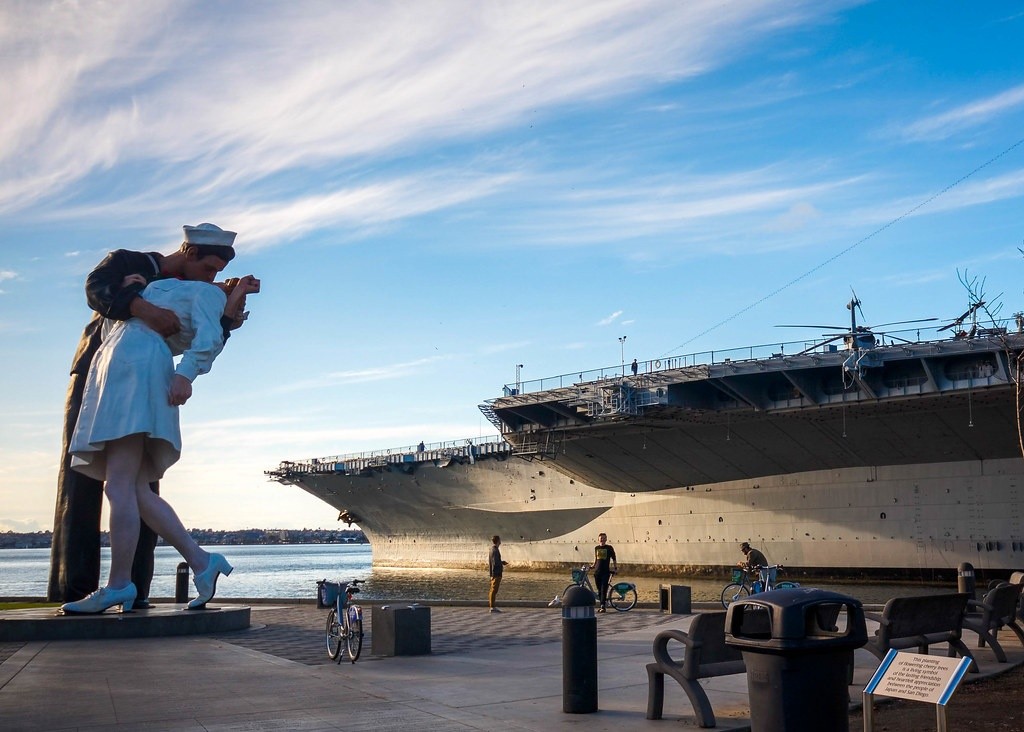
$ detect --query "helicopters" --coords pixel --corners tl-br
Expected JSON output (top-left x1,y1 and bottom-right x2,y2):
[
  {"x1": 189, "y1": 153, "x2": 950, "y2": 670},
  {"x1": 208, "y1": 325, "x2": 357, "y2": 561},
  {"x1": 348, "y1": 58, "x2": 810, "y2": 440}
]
[{"x1": 773, "y1": 283, "x2": 938, "y2": 355}]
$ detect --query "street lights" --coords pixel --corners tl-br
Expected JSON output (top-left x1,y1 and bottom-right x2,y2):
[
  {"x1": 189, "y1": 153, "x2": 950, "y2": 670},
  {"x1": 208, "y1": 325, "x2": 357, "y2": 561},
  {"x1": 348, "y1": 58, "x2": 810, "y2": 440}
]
[{"x1": 618, "y1": 336, "x2": 627, "y2": 376}]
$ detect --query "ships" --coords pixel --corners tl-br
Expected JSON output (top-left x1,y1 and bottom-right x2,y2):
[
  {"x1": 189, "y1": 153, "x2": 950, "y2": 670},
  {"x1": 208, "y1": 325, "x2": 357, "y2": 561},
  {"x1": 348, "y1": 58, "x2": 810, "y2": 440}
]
[{"x1": 262, "y1": 315, "x2": 1024, "y2": 586}]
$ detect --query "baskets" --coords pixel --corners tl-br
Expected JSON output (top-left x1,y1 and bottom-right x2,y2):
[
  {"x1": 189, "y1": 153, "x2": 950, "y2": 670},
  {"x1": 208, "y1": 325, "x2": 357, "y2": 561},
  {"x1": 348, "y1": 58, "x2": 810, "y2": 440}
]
[
  {"x1": 730, "y1": 567, "x2": 743, "y2": 582},
  {"x1": 321, "y1": 582, "x2": 349, "y2": 607},
  {"x1": 572, "y1": 567, "x2": 587, "y2": 583}
]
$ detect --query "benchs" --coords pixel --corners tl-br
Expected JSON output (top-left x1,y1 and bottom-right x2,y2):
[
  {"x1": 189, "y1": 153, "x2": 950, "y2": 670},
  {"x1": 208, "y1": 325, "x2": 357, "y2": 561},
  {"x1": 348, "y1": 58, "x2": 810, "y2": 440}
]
[
  {"x1": 645, "y1": 599, "x2": 855, "y2": 727},
  {"x1": 982, "y1": 572, "x2": 1024, "y2": 600},
  {"x1": 856, "y1": 591, "x2": 980, "y2": 673},
  {"x1": 964, "y1": 582, "x2": 1024, "y2": 663}
]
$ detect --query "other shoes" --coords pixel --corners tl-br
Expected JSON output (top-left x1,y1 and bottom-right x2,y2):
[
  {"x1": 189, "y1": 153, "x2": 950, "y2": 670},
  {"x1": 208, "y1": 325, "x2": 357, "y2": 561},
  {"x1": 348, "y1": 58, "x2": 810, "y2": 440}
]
[
  {"x1": 490, "y1": 606, "x2": 503, "y2": 613},
  {"x1": 598, "y1": 608, "x2": 606, "y2": 612}
]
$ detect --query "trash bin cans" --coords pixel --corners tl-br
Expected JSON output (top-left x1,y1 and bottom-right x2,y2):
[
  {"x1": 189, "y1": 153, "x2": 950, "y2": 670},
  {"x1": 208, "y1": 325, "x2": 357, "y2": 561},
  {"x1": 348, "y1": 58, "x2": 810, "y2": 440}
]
[
  {"x1": 725, "y1": 588, "x2": 869, "y2": 732},
  {"x1": 316, "y1": 581, "x2": 347, "y2": 609}
]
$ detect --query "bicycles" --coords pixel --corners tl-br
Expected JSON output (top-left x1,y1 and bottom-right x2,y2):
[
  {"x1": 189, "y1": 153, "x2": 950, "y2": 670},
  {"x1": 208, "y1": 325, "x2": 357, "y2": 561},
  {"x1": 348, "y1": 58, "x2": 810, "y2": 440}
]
[
  {"x1": 561, "y1": 565, "x2": 638, "y2": 612},
  {"x1": 316, "y1": 579, "x2": 367, "y2": 664},
  {"x1": 721, "y1": 565, "x2": 800, "y2": 611}
]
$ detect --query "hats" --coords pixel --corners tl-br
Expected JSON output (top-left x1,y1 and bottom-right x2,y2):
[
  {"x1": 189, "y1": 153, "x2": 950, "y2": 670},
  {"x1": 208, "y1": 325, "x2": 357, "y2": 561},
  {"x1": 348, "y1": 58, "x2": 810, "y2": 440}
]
[{"x1": 740, "y1": 542, "x2": 750, "y2": 551}]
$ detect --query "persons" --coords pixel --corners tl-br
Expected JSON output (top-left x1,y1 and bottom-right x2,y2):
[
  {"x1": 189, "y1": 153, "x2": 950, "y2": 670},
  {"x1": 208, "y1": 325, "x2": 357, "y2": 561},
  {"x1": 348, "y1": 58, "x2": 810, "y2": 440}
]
[
  {"x1": 488, "y1": 534, "x2": 509, "y2": 613},
  {"x1": 631, "y1": 359, "x2": 638, "y2": 377},
  {"x1": 736, "y1": 542, "x2": 768, "y2": 595},
  {"x1": 588, "y1": 533, "x2": 617, "y2": 613},
  {"x1": 47, "y1": 223, "x2": 260, "y2": 615}
]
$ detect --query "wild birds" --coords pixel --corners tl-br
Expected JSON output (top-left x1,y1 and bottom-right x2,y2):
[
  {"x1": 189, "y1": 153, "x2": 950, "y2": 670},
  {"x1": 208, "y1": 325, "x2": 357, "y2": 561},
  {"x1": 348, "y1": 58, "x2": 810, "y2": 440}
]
[{"x1": 548, "y1": 595, "x2": 560, "y2": 607}]
